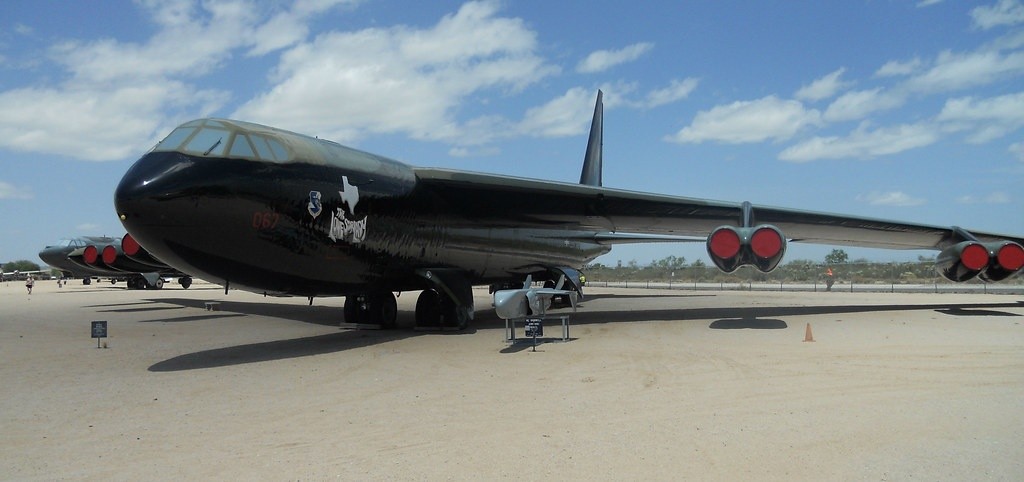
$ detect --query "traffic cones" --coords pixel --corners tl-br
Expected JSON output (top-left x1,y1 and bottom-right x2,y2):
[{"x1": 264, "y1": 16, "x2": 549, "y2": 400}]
[{"x1": 802, "y1": 322, "x2": 814, "y2": 342}]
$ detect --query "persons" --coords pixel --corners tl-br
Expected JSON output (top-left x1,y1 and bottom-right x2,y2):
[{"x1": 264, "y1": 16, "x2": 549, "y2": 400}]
[{"x1": 26, "y1": 276, "x2": 34, "y2": 294}]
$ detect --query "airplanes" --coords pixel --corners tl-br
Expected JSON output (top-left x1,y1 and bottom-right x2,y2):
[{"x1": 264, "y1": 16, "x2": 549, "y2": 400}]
[{"x1": 0, "y1": 89, "x2": 1024, "y2": 332}]
